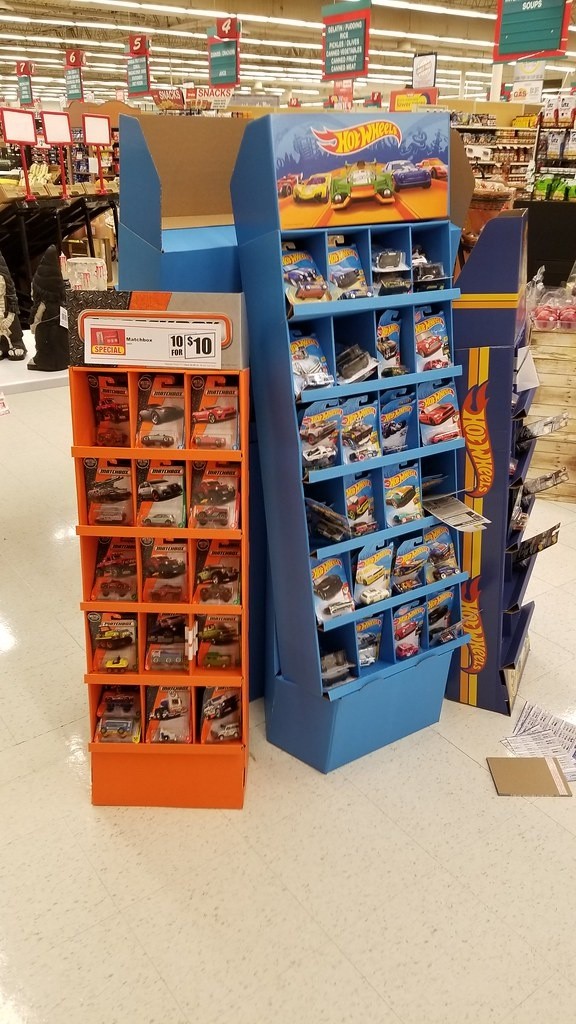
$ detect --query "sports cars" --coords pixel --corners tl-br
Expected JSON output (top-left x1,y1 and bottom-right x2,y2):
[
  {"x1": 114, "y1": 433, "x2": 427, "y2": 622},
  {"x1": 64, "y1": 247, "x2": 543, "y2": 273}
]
[
  {"x1": 193, "y1": 435, "x2": 227, "y2": 448},
  {"x1": 138, "y1": 403, "x2": 183, "y2": 424},
  {"x1": 140, "y1": 433, "x2": 173, "y2": 447},
  {"x1": 193, "y1": 405, "x2": 235, "y2": 424},
  {"x1": 293, "y1": 173, "x2": 331, "y2": 202}
]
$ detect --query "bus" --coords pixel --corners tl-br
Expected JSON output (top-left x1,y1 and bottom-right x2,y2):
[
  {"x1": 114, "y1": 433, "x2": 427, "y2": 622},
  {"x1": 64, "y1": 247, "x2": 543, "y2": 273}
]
[{"x1": 100, "y1": 720, "x2": 132, "y2": 737}]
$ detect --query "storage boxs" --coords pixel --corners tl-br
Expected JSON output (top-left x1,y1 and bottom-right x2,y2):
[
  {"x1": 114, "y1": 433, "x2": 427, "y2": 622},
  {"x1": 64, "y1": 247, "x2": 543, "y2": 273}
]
[
  {"x1": 1, "y1": 180, "x2": 120, "y2": 199},
  {"x1": 61, "y1": 211, "x2": 113, "y2": 282}
]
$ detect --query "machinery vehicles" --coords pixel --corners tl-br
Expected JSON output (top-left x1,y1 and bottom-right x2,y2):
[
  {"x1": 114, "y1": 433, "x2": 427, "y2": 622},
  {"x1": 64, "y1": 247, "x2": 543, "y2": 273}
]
[
  {"x1": 94, "y1": 508, "x2": 127, "y2": 524},
  {"x1": 88, "y1": 476, "x2": 130, "y2": 502}
]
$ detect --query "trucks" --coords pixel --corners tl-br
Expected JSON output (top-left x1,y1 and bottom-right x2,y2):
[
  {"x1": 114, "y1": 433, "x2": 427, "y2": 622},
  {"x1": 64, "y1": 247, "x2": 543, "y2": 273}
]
[
  {"x1": 203, "y1": 653, "x2": 232, "y2": 670},
  {"x1": 199, "y1": 622, "x2": 238, "y2": 644},
  {"x1": 148, "y1": 689, "x2": 187, "y2": 720}
]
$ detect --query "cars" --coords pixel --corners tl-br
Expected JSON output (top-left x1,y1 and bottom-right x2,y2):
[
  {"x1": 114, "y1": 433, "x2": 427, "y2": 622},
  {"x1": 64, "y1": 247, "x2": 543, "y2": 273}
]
[
  {"x1": 297, "y1": 280, "x2": 411, "y2": 302},
  {"x1": 352, "y1": 511, "x2": 422, "y2": 537},
  {"x1": 141, "y1": 514, "x2": 174, "y2": 527},
  {"x1": 278, "y1": 158, "x2": 448, "y2": 211},
  {"x1": 347, "y1": 483, "x2": 417, "y2": 519},
  {"x1": 291, "y1": 331, "x2": 443, "y2": 373},
  {"x1": 285, "y1": 247, "x2": 442, "y2": 298},
  {"x1": 105, "y1": 695, "x2": 135, "y2": 713},
  {"x1": 299, "y1": 401, "x2": 454, "y2": 446},
  {"x1": 311, "y1": 539, "x2": 451, "y2": 599},
  {"x1": 284, "y1": 263, "x2": 360, "y2": 288},
  {"x1": 325, "y1": 564, "x2": 459, "y2": 616},
  {"x1": 306, "y1": 359, "x2": 449, "y2": 388},
  {"x1": 96, "y1": 397, "x2": 129, "y2": 422},
  {"x1": 321, "y1": 628, "x2": 454, "y2": 688},
  {"x1": 100, "y1": 427, "x2": 126, "y2": 445},
  {"x1": 139, "y1": 479, "x2": 181, "y2": 502},
  {"x1": 301, "y1": 431, "x2": 462, "y2": 465},
  {"x1": 357, "y1": 603, "x2": 449, "y2": 649}
]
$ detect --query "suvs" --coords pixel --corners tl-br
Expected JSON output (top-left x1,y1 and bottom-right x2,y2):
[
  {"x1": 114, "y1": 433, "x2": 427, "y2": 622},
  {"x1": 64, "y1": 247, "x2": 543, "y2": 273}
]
[
  {"x1": 151, "y1": 584, "x2": 181, "y2": 602},
  {"x1": 96, "y1": 551, "x2": 136, "y2": 577},
  {"x1": 101, "y1": 579, "x2": 129, "y2": 598},
  {"x1": 210, "y1": 722, "x2": 239, "y2": 740},
  {"x1": 197, "y1": 506, "x2": 229, "y2": 527},
  {"x1": 201, "y1": 585, "x2": 232, "y2": 602},
  {"x1": 105, "y1": 655, "x2": 128, "y2": 674},
  {"x1": 193, "y1": 480, "x2": 236, "y2": 505},
  {"x1": 203, "y1": 694, "x2": 239, "y2": 720},
  {"x1": 144, "y1": 555, "x2": 185, "y2": 578},
  {"x1": 196, "y1": 563, "x2": 239, "y2": 585},
  {"x1": 94, "y1": 627, "x2": 133, "y2": 649}
]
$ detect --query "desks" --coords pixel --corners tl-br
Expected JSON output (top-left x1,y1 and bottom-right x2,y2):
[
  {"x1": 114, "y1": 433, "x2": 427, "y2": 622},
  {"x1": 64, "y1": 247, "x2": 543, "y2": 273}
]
[{"x1": 524, "y1": 328, "x2": 576, "y2": 506}]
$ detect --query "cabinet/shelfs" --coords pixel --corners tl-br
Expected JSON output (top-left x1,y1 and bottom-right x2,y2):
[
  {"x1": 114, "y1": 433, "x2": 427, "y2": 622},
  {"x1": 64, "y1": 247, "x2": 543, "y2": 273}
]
[
  {"x1": 444, "y1": 291, "x2": 571, "y2": 718},
  {"x1": 239, "y1": 219, "x2": 473, "y2": 775},
  {"x1": 0, "y1": 129, "x2": 120, "y2": 183},
  {"x1": 455, "y1": 122, "x2": 576, "y2": 207},
  {"x1": 67, "y1": 365, "x2": 248, "y2": 810}
]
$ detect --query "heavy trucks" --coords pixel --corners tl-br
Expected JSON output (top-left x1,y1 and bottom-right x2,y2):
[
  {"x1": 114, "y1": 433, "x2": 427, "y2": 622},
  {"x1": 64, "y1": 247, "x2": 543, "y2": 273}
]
[
  {"x1": 149, "y1": 650, "x2": 184, "y2": 665},
  {"x1": 149, "y1": 615, "x2": 185, "y2": 639}
]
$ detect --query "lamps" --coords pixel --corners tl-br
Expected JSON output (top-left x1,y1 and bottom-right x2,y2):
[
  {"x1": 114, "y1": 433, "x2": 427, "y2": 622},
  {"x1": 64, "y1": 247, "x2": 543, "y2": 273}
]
[{"x1": 0, "y1": 0, "x2": 576, "y2": 108}]
